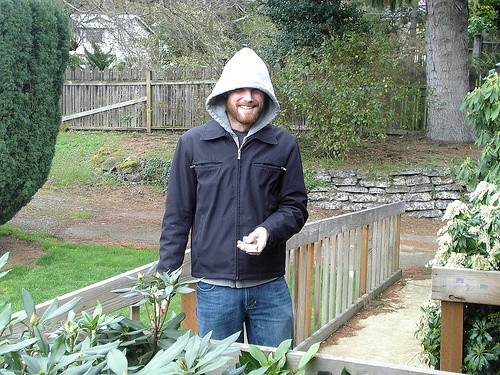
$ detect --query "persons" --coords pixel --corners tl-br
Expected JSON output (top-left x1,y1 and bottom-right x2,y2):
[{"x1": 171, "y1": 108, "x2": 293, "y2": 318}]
[{"x1": 151, "y1": 45, "x2": 310, "y2": 353}]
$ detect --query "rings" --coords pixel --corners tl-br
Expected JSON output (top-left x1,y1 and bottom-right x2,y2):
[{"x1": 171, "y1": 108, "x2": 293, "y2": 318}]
[{"x1": 255, "y1": 244, "x2": 259, "y2": 253}]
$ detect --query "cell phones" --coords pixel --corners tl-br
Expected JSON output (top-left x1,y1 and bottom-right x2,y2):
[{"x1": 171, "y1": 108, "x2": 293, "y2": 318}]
[{"x1": 242, "y1": 235, "x2": 259, "y2": 254}]
